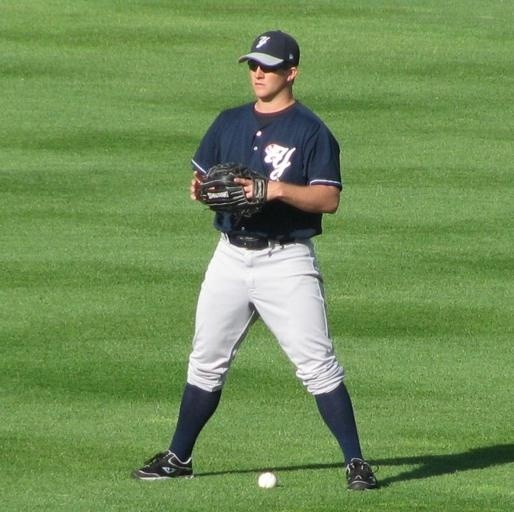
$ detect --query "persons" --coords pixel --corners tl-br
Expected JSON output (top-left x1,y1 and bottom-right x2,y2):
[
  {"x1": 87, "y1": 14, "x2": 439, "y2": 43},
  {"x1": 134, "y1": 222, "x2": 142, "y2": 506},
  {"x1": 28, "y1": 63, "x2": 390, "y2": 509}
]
[{"x1": 130, "y1": 31, "x2": 379, "y2": 490}]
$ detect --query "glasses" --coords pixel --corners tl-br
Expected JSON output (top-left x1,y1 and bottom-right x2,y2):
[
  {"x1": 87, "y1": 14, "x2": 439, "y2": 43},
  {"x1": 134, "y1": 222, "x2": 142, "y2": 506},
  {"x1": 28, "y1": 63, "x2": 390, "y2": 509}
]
[{"x1": 248, "y1": 59, "x2": 279, "y2": 74}]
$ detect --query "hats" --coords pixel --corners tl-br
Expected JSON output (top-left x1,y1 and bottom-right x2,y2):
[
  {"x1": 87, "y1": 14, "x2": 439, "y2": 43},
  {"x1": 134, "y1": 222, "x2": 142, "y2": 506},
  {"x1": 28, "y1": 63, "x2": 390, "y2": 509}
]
[{"x1": 238, "y1": 29, "x2": 301, "y2": 67}]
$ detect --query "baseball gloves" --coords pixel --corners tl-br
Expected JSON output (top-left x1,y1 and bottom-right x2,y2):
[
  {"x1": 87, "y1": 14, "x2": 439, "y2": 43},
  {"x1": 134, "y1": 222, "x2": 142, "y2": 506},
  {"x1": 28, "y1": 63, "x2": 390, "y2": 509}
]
[{"x1": 198, "y1": 163, "x2": 268, "y2": 214}]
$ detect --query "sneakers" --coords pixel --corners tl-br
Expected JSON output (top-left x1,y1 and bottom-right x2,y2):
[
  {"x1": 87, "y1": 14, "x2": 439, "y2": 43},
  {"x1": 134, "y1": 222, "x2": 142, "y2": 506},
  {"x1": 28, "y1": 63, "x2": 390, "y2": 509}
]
[
  {"x1": 130, "y1": 450, "x2": 194, "y2": 481},
  {"x1": 345, "y1": 458, "x2": 379, "y2": 490}
]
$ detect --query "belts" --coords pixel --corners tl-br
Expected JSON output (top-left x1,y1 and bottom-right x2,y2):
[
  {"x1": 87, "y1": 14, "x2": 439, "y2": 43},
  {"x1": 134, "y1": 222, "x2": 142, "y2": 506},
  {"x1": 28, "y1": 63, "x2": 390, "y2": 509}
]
[{"x1": 225, "y1": 234, "x2": 295, "y2": 250}]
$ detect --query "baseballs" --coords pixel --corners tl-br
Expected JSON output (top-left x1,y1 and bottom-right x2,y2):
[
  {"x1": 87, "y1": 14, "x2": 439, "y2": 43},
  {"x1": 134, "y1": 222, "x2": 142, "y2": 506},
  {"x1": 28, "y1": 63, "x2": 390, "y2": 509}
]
[{"x1": 258, "y1": 472, "x2": 277, "y2": 489}]
